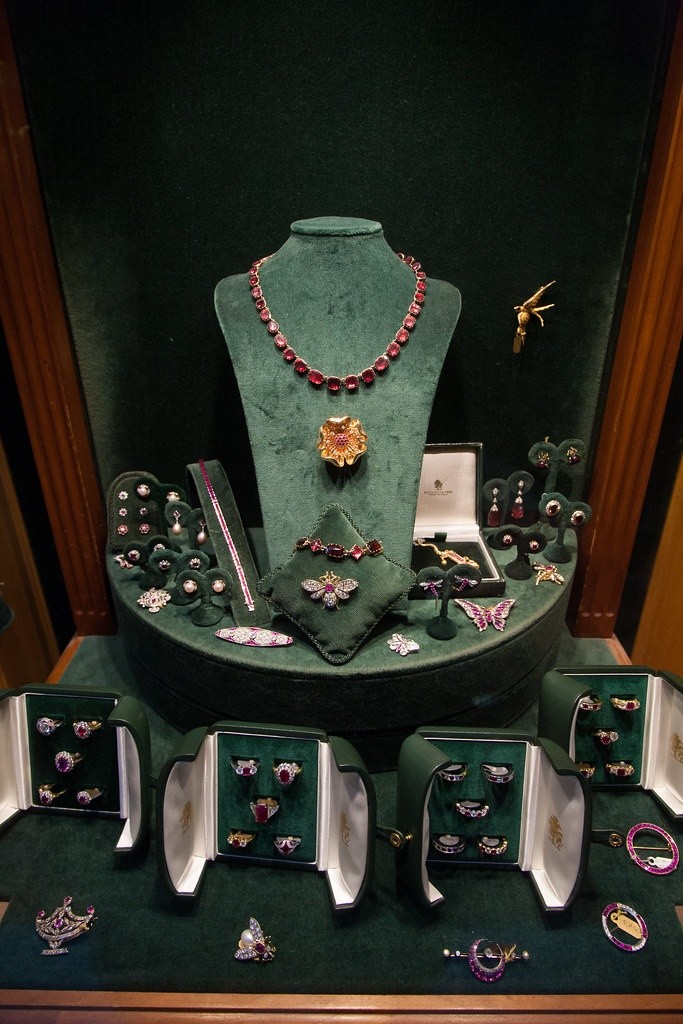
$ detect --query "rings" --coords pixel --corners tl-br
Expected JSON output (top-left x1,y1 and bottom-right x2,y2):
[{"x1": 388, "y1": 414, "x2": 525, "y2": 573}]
[
  {"x1": 274, "y1": 837, "x2": 301, "y2": 854},
  {"x1": 38, "y1": 718, "x2": 62, "y2": 736},
  {"x1": 274, "y1": 764, "x2": 301, "y2": 784},
  {"x1": 39, "y1": 784, "x2": 66, "y2": 803},
  {"x1": 480, "y1": 765, "x2": 514, "y2": 783},
  {"x1": 231, "y1": 760, "x2": 259, "y2": 775},
  {"x1": 227, "y1": 832, "x2": 253, "y2": 847},
  {"x1": 594, "y1": 731, "x2": 618, "y2": 744},
  {"x1": 576, "y1": 765, "x2": 594, "y2": 778},
  {"x1": 606, "y1": 764, "x2": 633, "y2": 776},
  {"x1": 432, "y1": 838, "x2": 465, "y2": 853},
  {"x1": 77, "y1": 790, "x2": 102, "y2": 805},
  {"x1": 54, "y1": 752, "x2": 82, "y2": 772},
  {"x1": 478, "y1": 838, "x2": 508, "y2": 855},
  {"x1": 438, "y1": 766, "x2": 468, "y2": 780},
  {"x1": 250, "y1": 798, "x2": 279, "y2": 823},
  {"x1": 72, "y1": 720, "x2": 101, "y2": 738},
  {"x1": 455, "y1": 803, "x2": 490, "y2": 817},
  {"x1": 580, "y1": 701, "x2": 603, "y2": 710},
  {"x1": 610, "y1": 698, "x2": 641, "y2": 710}
]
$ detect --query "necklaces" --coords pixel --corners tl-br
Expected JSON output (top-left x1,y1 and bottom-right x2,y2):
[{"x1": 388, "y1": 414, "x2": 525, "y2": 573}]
[{"x1": 250, "y1": 252, "x2": 426, "y2": 390}]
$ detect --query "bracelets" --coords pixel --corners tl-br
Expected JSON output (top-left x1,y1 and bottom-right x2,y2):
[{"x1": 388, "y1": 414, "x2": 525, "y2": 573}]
[
  {"x1": 296, "y1": 538, "x2": 382, "y2": 559},
  {"x1": 200, "y1": 460, "x2": 254, "y2": 614}
]
